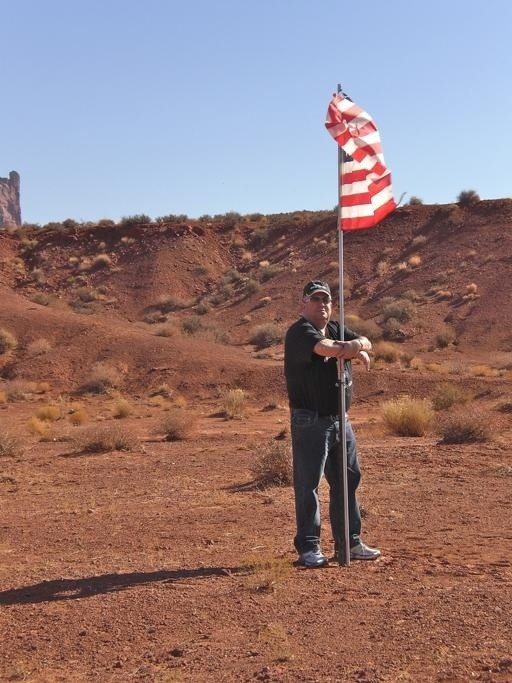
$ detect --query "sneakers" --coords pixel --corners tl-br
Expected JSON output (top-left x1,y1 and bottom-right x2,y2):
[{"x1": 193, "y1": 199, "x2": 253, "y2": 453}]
[
  {"x1": 298, "y1": 550, "x2": 328, "y2": 567},
  {"x1": 335, "y1": 542, "x2": 380, "y2": 560}
]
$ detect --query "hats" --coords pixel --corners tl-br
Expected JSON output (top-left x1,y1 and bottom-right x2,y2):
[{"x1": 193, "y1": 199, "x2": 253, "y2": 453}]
[{"x1": 302, "y1": 280, "x2": 331, "y2": 297}]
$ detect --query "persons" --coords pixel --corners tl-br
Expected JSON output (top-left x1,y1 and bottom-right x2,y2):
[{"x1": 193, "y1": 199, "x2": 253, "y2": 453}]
[{"x1": 284, "y1": 280, "x2": 381, "y2": 567}]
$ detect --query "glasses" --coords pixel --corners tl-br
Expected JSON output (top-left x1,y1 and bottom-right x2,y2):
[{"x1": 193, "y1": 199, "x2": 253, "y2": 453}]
[{"x1": 309, "y1": 296, "x2": 331, "y2": 304}]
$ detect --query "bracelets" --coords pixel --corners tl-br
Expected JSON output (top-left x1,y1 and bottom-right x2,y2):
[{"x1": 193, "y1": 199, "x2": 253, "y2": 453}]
[{"x1": 358, "y1": 339, "x2": 363, "y2": 349}]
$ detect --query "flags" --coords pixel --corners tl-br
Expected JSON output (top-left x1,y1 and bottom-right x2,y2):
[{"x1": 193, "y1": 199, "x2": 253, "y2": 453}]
[{"x1": 324, "y1": 89, "x2": 397, "y2": 231}]
